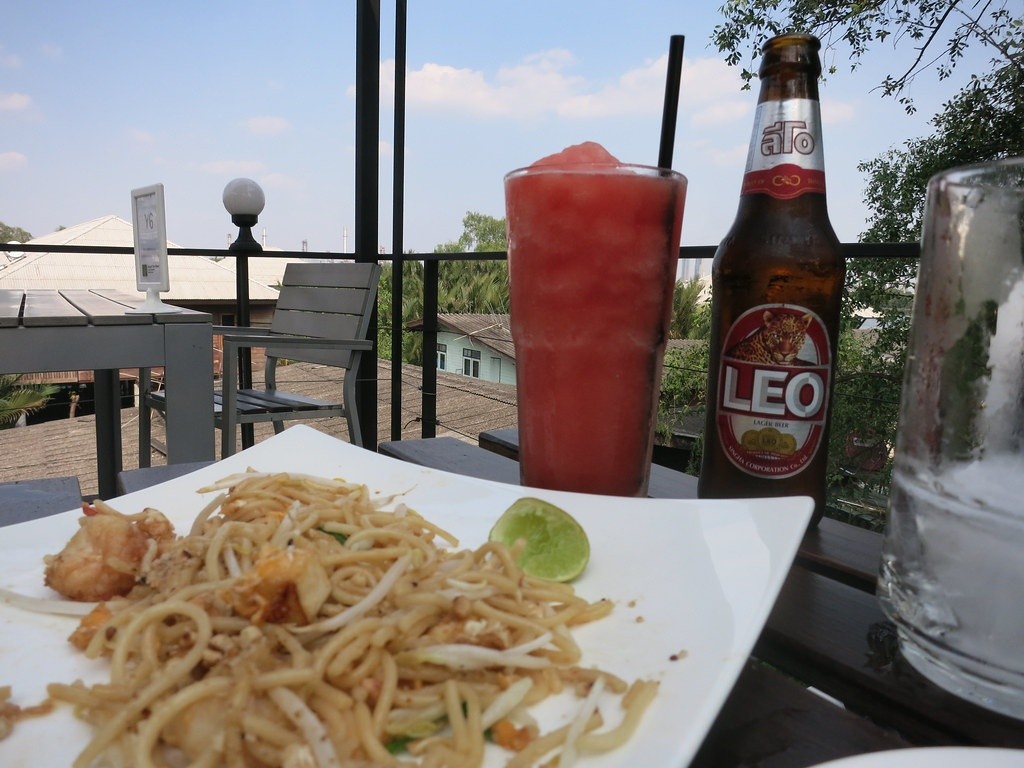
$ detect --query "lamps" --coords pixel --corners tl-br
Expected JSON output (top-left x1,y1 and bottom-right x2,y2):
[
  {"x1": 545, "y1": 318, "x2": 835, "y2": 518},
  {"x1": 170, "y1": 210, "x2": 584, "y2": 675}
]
[{"x1": 223, "y1": 179, "x2": 265, "y2": 251}]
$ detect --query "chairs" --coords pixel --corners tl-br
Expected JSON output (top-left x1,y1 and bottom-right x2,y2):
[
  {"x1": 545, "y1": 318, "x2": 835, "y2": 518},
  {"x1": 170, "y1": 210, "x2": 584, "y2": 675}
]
[{"x1": 139, "y1": 263, "x2": 383, "y2": 469}]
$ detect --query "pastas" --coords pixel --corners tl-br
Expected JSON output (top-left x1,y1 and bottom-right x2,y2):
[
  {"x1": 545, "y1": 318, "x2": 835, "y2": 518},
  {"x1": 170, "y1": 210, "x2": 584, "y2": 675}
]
[{"x1": 42, "y1": 472, "x2": 661, "y2": 768}]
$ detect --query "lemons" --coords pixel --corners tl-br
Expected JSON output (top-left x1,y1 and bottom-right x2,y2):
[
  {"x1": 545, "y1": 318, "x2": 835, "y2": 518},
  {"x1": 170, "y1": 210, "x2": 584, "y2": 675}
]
[{"x1": 488, "y1": 496, "x2": 591, "y2": 585}]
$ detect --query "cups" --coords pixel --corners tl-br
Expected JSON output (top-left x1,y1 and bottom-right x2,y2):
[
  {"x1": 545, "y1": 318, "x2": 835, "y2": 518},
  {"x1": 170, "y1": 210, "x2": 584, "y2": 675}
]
[
  {"x1": 504, "y1": 162, "x2": 689, "y2": 498},
  {"x1": 876, "y1": 156, "x2": 1023, "y2": 723}
]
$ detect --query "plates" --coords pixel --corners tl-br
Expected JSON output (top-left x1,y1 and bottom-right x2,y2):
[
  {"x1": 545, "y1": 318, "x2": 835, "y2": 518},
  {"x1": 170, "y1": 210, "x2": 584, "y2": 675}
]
[
  {"x1": 0, "y1": 424, "x2": 816, "y2": 768},
  {"x1": 805, "y1": 744, "x2": 1024, "y2": 768}
]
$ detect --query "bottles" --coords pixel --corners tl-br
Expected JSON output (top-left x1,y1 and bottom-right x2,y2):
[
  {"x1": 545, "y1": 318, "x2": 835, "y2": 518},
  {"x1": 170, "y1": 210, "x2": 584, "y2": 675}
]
[{"x1": 699, "y1": 34, "x2": 848, "y2": 533}]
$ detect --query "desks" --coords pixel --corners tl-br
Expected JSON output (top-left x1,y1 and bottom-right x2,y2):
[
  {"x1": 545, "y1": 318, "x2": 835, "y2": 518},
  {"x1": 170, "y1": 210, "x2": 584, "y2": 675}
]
[
  {"x1": 0, "y1": 289, "x2": 213, "y2": 506},
  {"x1": 1, "y1": 427, "x2": 1024, "y2": 768}
]
